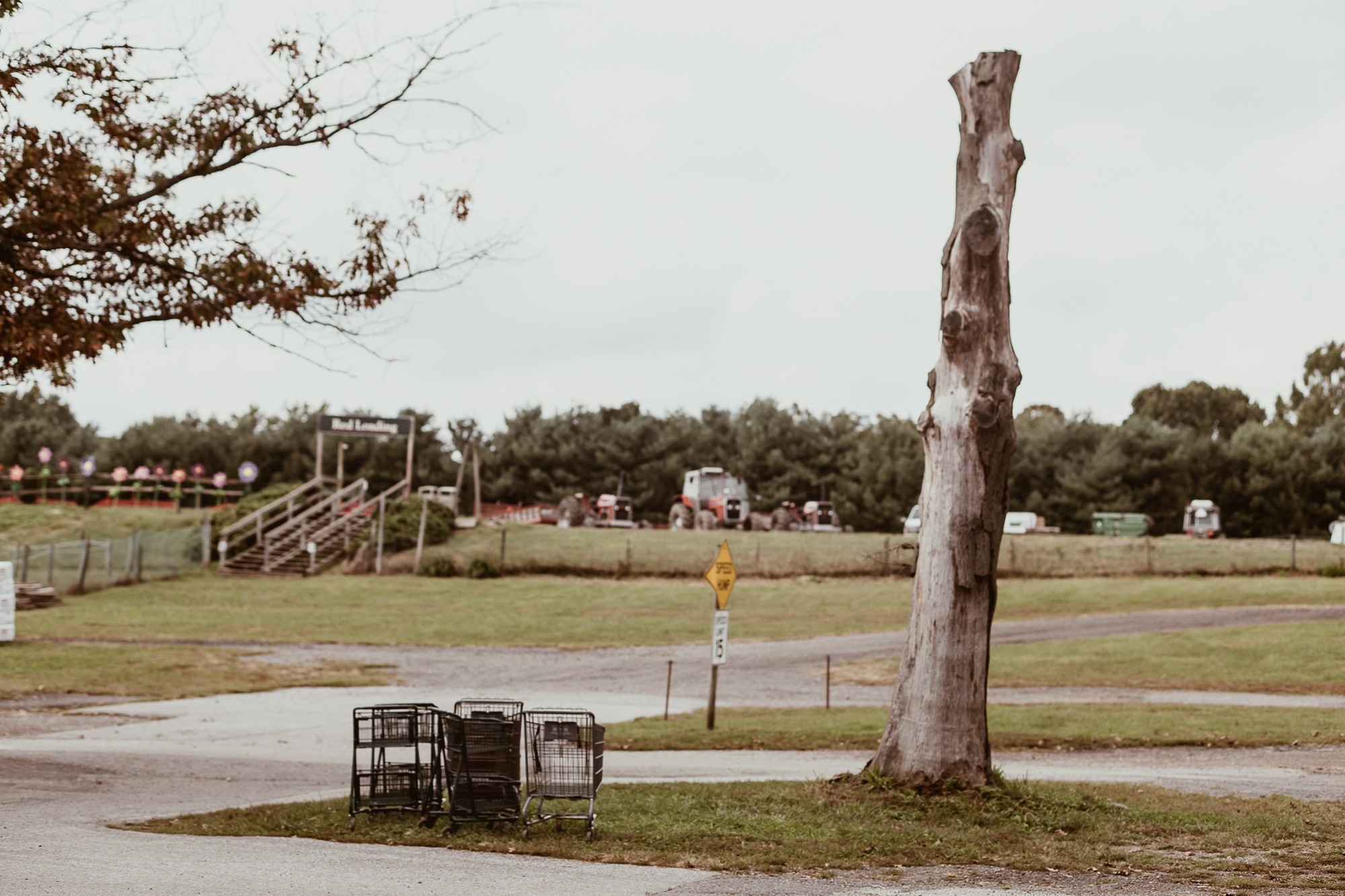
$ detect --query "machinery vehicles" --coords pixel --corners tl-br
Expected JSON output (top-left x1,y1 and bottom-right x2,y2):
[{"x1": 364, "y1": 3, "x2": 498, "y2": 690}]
[
  {"x1": 557, "y1": 492, "x2": 634, "y2": 529},
  {"x1": 669, "y1": 467, "x2": 752, "y2": 533}
]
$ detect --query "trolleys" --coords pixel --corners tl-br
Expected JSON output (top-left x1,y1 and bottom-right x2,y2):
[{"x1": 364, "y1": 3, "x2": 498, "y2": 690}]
[{"x1": 346, "y1": 698, "x2": 606, "y2": 844}]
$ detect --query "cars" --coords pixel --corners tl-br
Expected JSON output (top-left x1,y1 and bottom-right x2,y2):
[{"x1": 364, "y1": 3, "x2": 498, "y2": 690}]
[{"x1": 900, "y1": 503, "x2": 922, "y2": 533}]
